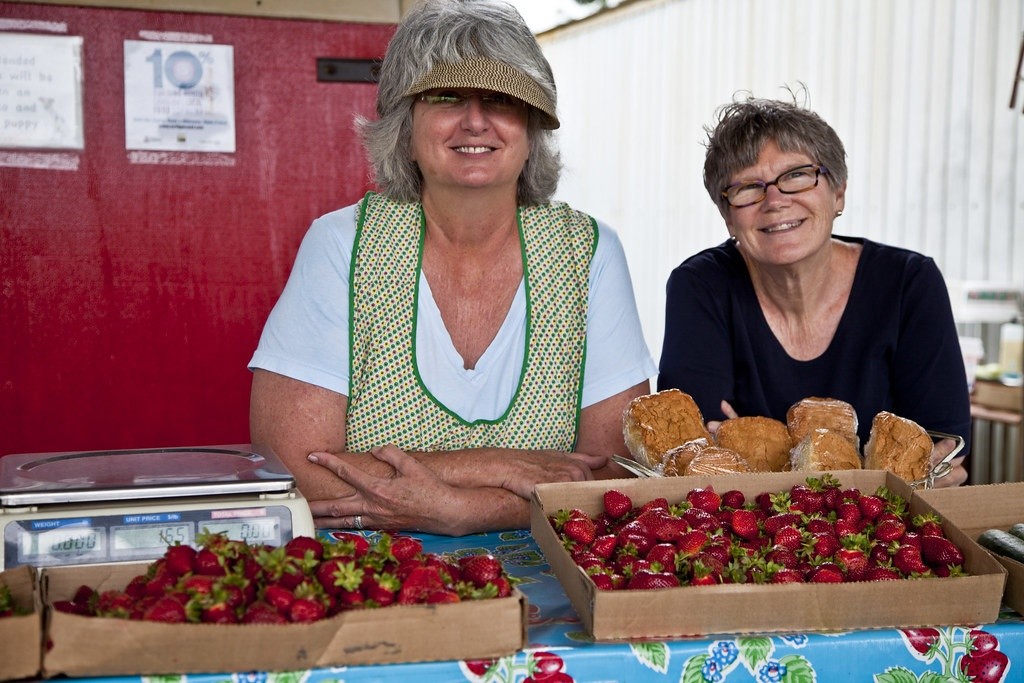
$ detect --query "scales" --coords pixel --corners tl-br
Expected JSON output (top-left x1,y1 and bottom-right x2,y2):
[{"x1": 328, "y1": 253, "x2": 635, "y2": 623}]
[{"x1": 0, "y1": 442, "x2": 316, "y2": 572}]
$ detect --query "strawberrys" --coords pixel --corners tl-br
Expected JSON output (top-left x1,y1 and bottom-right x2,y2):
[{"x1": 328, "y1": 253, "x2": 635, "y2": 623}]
[
  {"x1": 0, "y1": 527, "x2": 522, "y2": 625},
  {"x1": 547, "y1": 472, "x2": 973, "y2": 592}
]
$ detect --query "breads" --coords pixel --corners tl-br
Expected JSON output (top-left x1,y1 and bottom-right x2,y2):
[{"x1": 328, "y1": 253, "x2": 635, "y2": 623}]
[{"x1": 622, "y1": 387, "x2": 935, "y2": 489}]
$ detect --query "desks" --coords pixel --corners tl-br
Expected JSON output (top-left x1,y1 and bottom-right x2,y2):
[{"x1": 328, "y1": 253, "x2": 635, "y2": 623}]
[{"x1": 39, "y1": 526, "x2": 1024, "y2": 683}]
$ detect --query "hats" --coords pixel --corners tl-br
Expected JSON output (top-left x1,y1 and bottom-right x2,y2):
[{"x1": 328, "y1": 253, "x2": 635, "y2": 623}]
[{"x1": 397, "y1": 31, "x2": 560, "y2": 129}]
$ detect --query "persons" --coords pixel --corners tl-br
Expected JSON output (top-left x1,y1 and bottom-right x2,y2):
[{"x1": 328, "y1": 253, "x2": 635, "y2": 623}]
[
  {"x1": 248, "y1": 0, "x2": 658, "y2": 538},
  {"x1": 655, "y1": 81, "x2": 974, "y2": 489}
]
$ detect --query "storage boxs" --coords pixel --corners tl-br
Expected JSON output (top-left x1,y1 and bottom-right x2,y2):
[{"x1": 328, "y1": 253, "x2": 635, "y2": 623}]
[
  {"x1": 913, "y1": 482, "x2": 1024, "y2": 618},
  {"x1": 40, "y1": 559, "x2": 529, "y2": 677},
  {"x1": 0, "y1": 564, "x2": 47, "y2": 681},
  {"x1": 529, "y1": 468, "x2": 1009, "y2": 640}
]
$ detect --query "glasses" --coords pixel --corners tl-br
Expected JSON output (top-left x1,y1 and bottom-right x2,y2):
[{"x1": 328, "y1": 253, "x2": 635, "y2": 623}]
[
  {"x1": 721, "y1": 164, "x2": 830, "y2": 208},
  {"x1": 417, "y1": 88, "x2": 528, "y2": 115}
]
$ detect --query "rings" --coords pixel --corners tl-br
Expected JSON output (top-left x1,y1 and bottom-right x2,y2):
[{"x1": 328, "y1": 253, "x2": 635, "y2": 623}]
[{"x1": 353, "y1": 515, "x2": 364, "y2": 530}]
